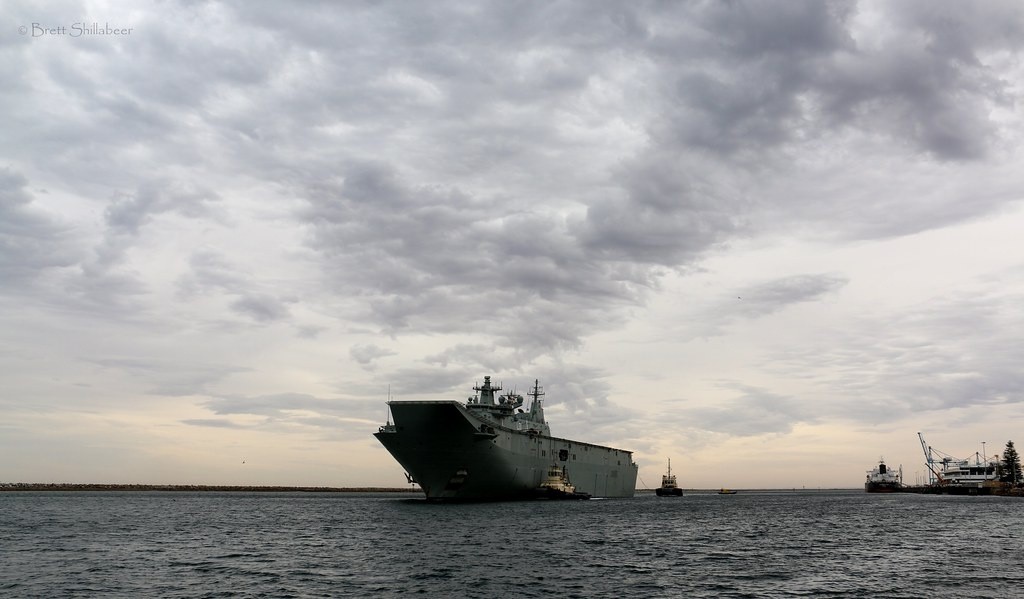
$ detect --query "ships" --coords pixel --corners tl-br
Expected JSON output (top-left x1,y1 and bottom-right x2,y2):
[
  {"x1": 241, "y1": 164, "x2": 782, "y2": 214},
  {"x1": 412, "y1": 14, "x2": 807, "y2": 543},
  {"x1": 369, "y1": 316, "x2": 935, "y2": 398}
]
[{"x1": 374, "y1": 376, "x2": 639, "y2": 501}]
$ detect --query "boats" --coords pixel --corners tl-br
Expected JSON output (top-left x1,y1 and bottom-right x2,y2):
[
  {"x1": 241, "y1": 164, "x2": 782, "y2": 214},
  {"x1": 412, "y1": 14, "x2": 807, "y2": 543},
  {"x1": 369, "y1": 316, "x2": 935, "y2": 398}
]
[
  {"x1": 718, "y1": 490, "x2": 737, "y2": 494},
  {"x1": 535, "y1": 463, "x2": 592, "y2": 500},
  {"x1": 655, "y1": 458, "x2": 684, "y2": 497},
  {"x1": 865, "y1": 455, "x2": 900, "y2": 493}
]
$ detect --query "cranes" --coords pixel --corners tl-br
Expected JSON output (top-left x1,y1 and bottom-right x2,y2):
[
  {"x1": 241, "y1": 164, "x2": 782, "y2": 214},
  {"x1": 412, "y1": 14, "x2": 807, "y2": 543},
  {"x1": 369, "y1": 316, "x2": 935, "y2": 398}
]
[
  {"x1": 925, "y1": 463, "x2": 948, "y2": 486},
  {"x1": 917, "y1": 432, "x2": 933, "y2": 463}
]
